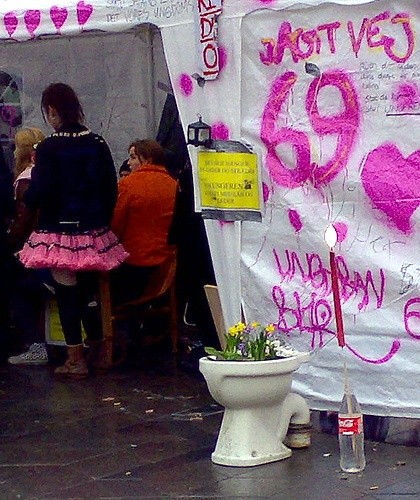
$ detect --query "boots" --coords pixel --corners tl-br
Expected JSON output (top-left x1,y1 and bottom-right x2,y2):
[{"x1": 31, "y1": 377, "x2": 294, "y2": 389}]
[
  {"x1": 8, "y1": 343, "x2": 48, "y2": 364},
  {"x1": 54, "y1": 344, "x2": 88, "y2": 373},
  {"x1": 85, "y1": 340, "x2": 100, "y2": 360}
]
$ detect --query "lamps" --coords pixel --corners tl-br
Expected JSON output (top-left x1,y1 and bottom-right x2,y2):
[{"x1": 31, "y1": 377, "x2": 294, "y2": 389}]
[{"x1": 187, "y1": 113, "x2": 213, "y2": 148}]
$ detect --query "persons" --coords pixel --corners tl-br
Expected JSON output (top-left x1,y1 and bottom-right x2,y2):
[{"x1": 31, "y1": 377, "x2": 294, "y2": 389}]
[
  {"x1": 119, "y1": 159, "x2": 131, "y2": 177},
  {"x1": 110, "y1": 138, "x2": 179, "y2": 305},
  {"x1": 23, "y1": 82, "x2": 129, "y2": 373},
  {"x1": 7, "y1": 128, "x2": 48, "y2": 366}
]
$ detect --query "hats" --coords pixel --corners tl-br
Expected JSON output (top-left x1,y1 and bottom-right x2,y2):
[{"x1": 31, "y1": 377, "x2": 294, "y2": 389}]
[{"x1": 119, "y1": 159, "x2": 131, "y2": 174}]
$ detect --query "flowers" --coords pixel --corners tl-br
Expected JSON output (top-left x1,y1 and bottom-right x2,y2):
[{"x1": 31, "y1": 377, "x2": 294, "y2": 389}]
[{"x1": 204, "y1": 321, "x2": 286, "y2": 362}]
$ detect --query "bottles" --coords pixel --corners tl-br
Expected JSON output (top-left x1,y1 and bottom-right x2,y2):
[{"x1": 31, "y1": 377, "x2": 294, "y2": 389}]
[{"x1": 338, "y1": 384, "x2": 366, "y2": 473}]
